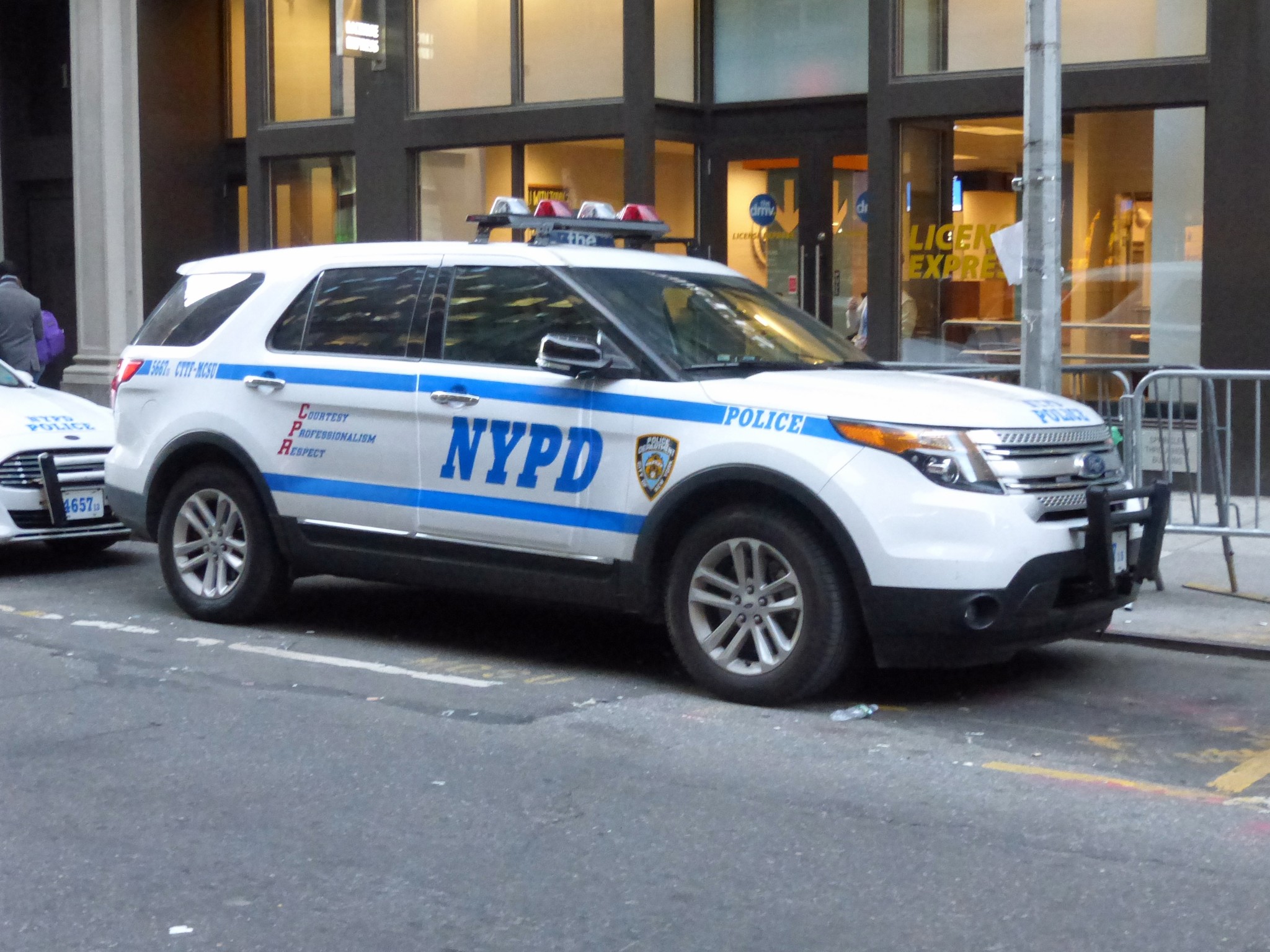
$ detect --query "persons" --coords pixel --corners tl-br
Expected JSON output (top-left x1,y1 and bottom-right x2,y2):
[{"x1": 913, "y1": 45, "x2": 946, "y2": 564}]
[
  {"x1": 844, "y1": 289, "x2": 918, "y2": 349},
  {"x1": 0, "y1": 260, "x2": 44, "y2": 373}
]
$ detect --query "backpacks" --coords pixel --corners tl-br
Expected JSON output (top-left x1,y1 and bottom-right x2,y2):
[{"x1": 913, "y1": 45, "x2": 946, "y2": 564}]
[{"x1": 36, "y1": 309, "x2": 67, "y2": 365}]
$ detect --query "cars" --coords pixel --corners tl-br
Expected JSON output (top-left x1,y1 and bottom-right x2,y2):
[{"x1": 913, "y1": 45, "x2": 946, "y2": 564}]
[{"x1": 0, "y1": 359, "x2": 136, "y2": 552}]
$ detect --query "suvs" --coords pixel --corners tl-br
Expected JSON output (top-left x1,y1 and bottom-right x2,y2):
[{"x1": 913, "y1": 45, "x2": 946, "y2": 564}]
[{"x1": 100, "y1": 199, "x2": 1173, "y2": 708}]
